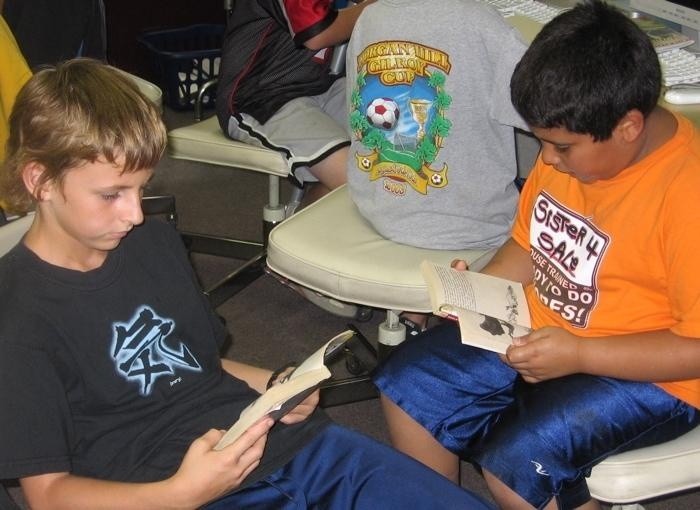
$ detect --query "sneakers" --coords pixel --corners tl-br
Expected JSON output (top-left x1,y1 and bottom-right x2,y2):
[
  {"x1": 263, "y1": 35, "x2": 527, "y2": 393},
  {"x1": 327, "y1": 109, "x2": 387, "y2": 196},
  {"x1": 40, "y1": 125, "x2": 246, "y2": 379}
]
[{"x1": 399, "y1": 317, "x2": 428, "y2": 337}]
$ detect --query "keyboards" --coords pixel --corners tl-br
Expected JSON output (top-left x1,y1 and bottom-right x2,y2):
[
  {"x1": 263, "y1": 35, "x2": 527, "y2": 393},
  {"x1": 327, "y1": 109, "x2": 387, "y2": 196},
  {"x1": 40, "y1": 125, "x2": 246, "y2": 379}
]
[
  {"x1": 488, "y1": 0, "x2": 556, "y2": 24},
  {"x1": 655, "y1": 48, "x2": 700, "y2": 86}
]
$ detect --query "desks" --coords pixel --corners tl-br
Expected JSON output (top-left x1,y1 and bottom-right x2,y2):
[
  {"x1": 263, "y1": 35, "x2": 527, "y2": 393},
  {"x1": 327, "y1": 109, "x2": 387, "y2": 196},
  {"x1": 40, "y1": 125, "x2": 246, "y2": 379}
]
[{"x1": 491, "y1": 0, "x2": 700, "y2": 180}]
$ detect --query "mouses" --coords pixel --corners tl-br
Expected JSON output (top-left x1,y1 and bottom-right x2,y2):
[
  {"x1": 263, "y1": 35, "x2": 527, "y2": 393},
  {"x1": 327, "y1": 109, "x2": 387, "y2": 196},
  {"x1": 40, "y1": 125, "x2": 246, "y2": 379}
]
[{"x1": 664, "y1": 84, "x2": 700, "y2": 105}]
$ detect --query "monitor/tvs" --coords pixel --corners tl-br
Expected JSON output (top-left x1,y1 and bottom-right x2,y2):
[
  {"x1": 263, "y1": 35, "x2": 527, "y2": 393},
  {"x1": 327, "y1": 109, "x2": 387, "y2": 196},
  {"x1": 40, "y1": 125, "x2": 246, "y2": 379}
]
[{"x1": 629, "y1": 0, "x2": 700, "y2": 31}]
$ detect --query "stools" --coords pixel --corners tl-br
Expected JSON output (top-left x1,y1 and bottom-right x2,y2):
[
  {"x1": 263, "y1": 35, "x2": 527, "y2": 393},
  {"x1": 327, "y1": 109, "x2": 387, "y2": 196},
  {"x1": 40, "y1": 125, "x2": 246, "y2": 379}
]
[
  {"x1": 267, "y1": 182, "x2": 497, "y2": 408},
  {"x1": 168, "y1": 115, "x2": 303, "y2": 308},
  {"x1": 585, "y1": 424, "x2": 700, "y2": 510}
]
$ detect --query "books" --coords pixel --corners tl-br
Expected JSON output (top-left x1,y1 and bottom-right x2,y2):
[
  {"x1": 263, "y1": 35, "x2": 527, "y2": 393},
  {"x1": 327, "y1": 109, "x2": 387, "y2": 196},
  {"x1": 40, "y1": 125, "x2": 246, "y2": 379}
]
[
  {"x1": 419, "y1": 260, "x2": 532, "y2": 355},
  {"x1": 212, "y1": 330, "x2": 354, "y2": 449},
  {"x1": 630, "y1": 18, "x2": 694, "y2": 54}
]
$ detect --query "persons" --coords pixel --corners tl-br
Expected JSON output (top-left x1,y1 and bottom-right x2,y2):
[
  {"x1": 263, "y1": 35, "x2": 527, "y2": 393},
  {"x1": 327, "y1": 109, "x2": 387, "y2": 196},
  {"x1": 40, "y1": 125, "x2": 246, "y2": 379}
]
[
  {"x1": 216, "y1": 3, "x2": 367, "y2": 216},
  {"x1": 345, "y1": 2, "x2": 533, "y2": 341},
  {"x1": 1, "y1": 57, "x2": 495, "y2": 509},
  {"x1": 369, "y1": 2, "x2": 700, "y2": 509}
]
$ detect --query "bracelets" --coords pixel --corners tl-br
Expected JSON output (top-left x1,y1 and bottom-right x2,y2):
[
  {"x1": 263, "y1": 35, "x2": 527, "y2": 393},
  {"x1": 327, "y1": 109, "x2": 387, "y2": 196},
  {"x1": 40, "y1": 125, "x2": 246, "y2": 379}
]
[{"x1": 266, "y1": 367, "x2": 285, "y2": 391}]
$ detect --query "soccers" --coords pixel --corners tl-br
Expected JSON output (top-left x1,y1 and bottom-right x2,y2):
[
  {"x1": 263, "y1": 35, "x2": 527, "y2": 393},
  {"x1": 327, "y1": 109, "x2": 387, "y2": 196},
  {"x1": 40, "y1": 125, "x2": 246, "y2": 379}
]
[{"x1": 365, "y1": 97, "x2": 400, "y2": 129}]
